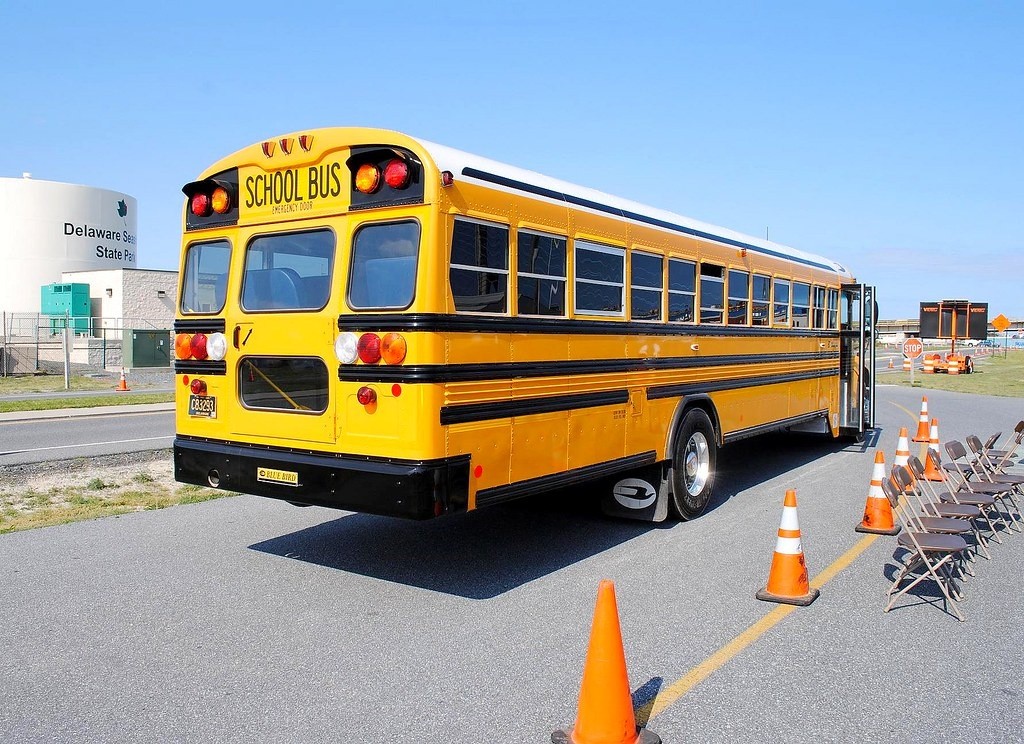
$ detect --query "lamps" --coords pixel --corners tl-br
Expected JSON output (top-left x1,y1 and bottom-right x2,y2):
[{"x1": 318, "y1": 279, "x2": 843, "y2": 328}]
[
  {"x1": 106, "y1": 288, "x2": 112, "y2": 295},
  {"x1": 158, "y1": 290, "x2": 165, "y2": 298}
]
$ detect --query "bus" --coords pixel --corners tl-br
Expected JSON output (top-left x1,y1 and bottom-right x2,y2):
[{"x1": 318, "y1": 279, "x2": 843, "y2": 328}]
[{"x1": 170, "y1": 126, "x2": 881, "y2": 524}]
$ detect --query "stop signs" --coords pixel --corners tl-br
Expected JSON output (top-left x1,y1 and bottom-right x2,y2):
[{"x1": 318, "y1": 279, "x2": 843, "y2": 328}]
[{"x1": 902, "y1": 339, "x2": 923, "y2": 359}]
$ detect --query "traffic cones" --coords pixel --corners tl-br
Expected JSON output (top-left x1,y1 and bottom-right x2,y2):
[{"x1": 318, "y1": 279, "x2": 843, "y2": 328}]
[
  {"x1": 911, "y1": 396, "x2": 931, "y2": 443},
  {"x1": 903, "y1": 359, "x2": 910, "y2": 371},
  {"x1": 756, "y1": 489, "x2": 821, "y2": 607},
  {"x1": 921, "y1": 418, "x2": 949, "y2": 482},
  {"x1": 887, "y1": 357, "x2": 894, "y2": 368},
  {"x1": 855, "y1": 451, "x2": 902, "y2": 537},
  {"x1": 948, "y1": 358, "x2": 958, "y2": 375},
  {"x1": 924, "y1": 357, "x2": 934, "y2": 374},
  {"x1": 890, "y1": 427, "x2": 921, "y2": 496},
  {"x1": 549, "y1": 579, "x2": 668, "y2": 744},
  {"x1": 116, "y1": 367, "x2": 130, "y2": 391}
]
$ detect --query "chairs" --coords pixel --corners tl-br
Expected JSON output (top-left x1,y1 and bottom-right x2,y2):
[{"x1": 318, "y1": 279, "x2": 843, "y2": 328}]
[
  {"x1": 881, "y1": 422, "x2": 1024, "y2": 621},
  {"x1": 215, "y1": 267, "x2": 305, "y2": 310},
  {"x1": 351, "y1": 256, "x2": 417, "y2": 307}
]
